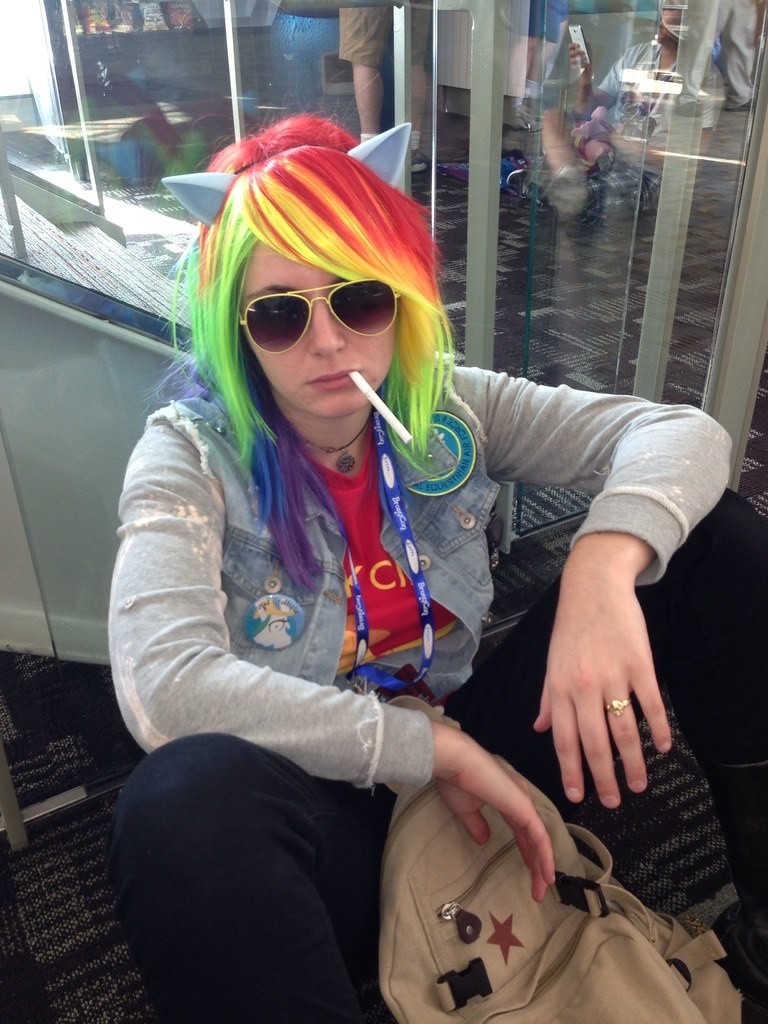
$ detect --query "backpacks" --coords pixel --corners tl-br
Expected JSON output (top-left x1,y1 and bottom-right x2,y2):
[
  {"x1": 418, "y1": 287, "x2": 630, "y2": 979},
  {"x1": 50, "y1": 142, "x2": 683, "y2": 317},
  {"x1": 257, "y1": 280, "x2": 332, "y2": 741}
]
[{"x1": 378, "y1": 695, "x2": 742, "y2": 1024}]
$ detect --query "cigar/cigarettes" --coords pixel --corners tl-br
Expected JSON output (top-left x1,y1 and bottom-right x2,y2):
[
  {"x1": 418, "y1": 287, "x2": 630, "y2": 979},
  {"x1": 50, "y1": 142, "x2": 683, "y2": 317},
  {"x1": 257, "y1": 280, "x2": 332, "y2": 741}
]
[{"x1": 349, "y1": 371, "x2": 414, "y2": 445}]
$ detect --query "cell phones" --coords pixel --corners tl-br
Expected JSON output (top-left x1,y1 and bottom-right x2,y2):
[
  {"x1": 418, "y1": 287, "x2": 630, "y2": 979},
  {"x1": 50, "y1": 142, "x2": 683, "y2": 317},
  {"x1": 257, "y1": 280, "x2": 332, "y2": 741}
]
[{"x1": 568, "y1": 25, "x2": 592, "y2": 64}]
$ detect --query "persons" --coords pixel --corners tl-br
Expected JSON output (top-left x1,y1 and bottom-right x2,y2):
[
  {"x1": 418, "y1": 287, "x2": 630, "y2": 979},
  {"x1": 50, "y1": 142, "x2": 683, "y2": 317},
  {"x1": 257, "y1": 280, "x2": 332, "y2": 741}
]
[
  {"x1": 108, "y1": 118, "x2": 768, "y2": 1024},
  {"x1": 337, "y1": 0, "x2": 432, "y2": 173},
  {"x1": 500, "y1": 0, "x2": 757, "y2": 226}
]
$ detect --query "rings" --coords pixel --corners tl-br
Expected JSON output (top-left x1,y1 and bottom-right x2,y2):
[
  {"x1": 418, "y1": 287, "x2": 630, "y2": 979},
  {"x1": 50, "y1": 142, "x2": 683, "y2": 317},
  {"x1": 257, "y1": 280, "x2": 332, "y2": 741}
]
[{"x1": 607, "y1": 699, "x2": 630, "y2": 717}]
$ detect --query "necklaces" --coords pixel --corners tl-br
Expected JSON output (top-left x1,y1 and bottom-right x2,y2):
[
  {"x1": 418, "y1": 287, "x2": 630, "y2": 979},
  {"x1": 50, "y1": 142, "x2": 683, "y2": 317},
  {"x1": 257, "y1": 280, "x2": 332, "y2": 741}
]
[{"x1": 297, "y1": 408, "x2": 375, "y2": 474}]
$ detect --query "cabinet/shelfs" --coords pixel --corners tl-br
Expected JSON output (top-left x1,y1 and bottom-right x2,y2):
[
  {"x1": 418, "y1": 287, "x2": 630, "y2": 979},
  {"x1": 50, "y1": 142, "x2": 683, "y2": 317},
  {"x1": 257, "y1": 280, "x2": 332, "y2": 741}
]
[{"x1": 25, "y1": 0, "x2": 274, "y2": 181}]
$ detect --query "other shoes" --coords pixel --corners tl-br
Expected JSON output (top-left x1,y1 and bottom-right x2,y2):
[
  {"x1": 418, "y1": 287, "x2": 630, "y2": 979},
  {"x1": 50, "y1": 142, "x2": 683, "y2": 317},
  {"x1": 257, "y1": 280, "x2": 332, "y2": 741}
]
[
  {"x1": 512, "y1": 97, "x2": 542, "y2": 131},
  {"x1": 726, "y1": 98, "x2": 752, "y2": 111},
  {"x1": 411, "y1": 149, "x2": 426, "y2": 172}
]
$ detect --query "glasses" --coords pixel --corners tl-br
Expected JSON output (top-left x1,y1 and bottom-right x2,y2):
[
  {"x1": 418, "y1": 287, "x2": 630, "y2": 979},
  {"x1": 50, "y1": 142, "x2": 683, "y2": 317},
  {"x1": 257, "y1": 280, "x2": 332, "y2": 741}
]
[{"x1": 236, "y1": 279, "x2": 402, "y2": 353}]
